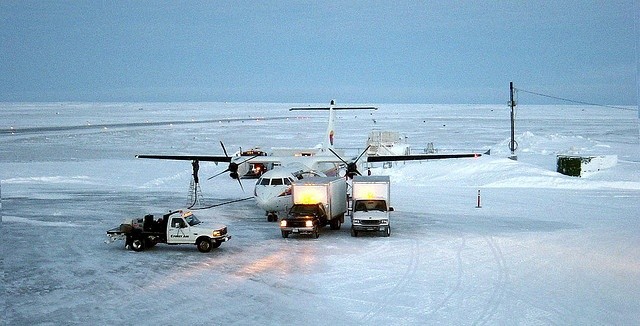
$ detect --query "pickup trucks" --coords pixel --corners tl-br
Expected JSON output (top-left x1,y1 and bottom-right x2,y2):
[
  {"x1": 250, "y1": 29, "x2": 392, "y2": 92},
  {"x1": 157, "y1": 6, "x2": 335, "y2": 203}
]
[{"x1": 107, "y1": 210, "x2": 232, "y2": 253}]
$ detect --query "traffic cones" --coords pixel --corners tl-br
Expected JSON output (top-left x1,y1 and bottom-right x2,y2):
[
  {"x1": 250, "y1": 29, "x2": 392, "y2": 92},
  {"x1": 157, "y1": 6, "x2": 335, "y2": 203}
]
[{"x1": 473, "y1": 188, "x2": 486, "y2": 209}]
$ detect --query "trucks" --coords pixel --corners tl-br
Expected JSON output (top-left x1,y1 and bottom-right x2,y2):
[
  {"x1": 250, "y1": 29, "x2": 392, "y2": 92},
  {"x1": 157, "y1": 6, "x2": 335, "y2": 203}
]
[
  {"x1": 280, "y1": 177, "x2": 347, "y2": 238},
  {"x1": 348, "y1": 176, "x2": 394, "y2": 237},
  {"x1": 235, "y1": 148, "x2": 275, "y2": 179}
]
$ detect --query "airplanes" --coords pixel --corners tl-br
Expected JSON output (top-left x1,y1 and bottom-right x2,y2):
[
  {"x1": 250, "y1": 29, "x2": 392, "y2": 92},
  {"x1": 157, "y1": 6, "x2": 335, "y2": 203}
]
[{"x1": 136, "y1": 97, "x2": 484, "y2": 224}]
